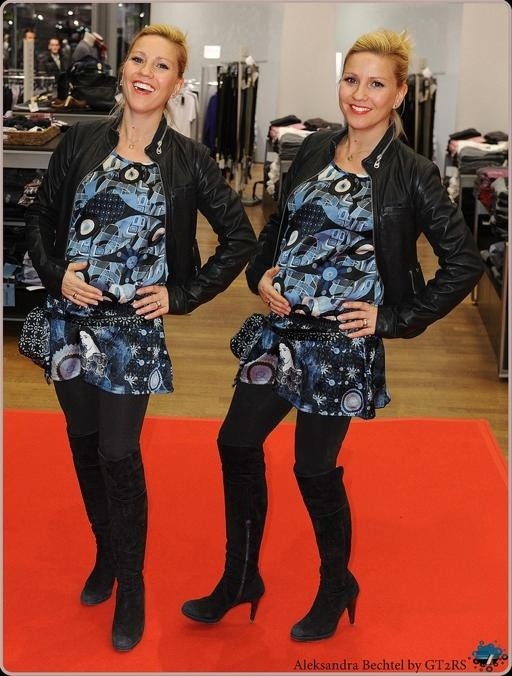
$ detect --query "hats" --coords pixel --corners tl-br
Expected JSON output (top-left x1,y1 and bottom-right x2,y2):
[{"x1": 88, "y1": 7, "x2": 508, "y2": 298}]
[{"x1": 91, "y1": 31, "x2": 108, "y2": 51}]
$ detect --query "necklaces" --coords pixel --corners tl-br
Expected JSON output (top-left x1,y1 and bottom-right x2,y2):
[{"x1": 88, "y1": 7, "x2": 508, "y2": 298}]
[
  {"x1": 117, "y1": 125, "x2": 160, "y2": 151},
  {"x1": 340, "y1": 135, "x2": 372, "y2": 161}
]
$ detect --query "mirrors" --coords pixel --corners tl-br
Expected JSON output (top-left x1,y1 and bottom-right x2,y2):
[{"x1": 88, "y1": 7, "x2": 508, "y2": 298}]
[{"x1": 3, "y1": 3, "x2": 151, "y2": 127}]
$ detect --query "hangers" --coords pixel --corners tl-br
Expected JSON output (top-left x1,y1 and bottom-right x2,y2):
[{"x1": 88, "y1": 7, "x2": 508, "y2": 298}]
[
  {"x1": 216, "y1": 61, "x2": 255, "y2": 86},
  {"x1": 170, "y1": 79, "x2": 193, "y2": 102}
]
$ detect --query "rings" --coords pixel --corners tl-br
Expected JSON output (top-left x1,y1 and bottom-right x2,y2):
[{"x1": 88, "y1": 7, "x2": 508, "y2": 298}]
[
  {"x1": 157, "y1": 301, "x2": 162, "y2": 309},
  {"x1": 362, "y1": 318, "x2": 368, "y2": 329},
  {"x1": 72, "y1": 292, "x2": 78, "y2": 298},
  {"x1": 266, "y1": 300, "x2": 271, "y2": 306}
]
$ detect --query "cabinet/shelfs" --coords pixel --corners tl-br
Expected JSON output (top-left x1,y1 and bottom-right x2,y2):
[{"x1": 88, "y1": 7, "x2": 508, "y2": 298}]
[
  {"x1": 3, "y1": 168, "x2": 53, "y2": 337},
  {"x1": 441, "y1": 153, "x2": 508, "y2": 383},
  {"x1": 262, "y1": 139, "x2": 294, "y2": 223}
]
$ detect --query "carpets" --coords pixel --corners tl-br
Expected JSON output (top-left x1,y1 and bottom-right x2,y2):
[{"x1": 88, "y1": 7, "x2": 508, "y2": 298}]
[{"x1": 2, "y1": 408, "x2": 508, "y2": 672}]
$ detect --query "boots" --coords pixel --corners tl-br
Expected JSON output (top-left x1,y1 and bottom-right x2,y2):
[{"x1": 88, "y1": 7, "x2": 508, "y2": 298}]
[
  {"x1": 64, "y1": 426, "x2": 148, "y2": 653},
  {"x1": 182, "y1": 439, "x2": 268, "y2": 624},
  {"x1": 289, "y1": 463, "x2": 360, "y2": 643}
]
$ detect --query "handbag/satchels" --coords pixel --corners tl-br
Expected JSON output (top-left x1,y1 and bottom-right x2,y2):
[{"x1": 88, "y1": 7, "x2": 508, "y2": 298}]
[
  {"x1": 58, "y1": 55, "x2": 118, "y2": 110},
  {"x1": 230, "y1": 311, "x2": 266, "y2": 358},
  {"x1": 18, "y1": 306, "x2": 51, "y2": 360}
]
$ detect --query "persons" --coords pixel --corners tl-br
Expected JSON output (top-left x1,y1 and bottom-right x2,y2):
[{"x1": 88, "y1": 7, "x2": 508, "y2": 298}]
[
  {"x1": 70, "y1": 29, "x2": 100, "y2": 60},
  {"x1": 17, "y1": 25, "x2": 264, "y2": 651},
  {"x1": 179, "y1": 28, "x2": 488, "y2": 642},
  {"x1": 35, "y1": 35, "x2": 72, "y2": 90},
  {"x1": 115, "y1": 27, "x2": 133, "y2": 66},
  {"x1": 15, "y1": 25, "x2": 45, "y2": 69},
  {"x1": 90, "y1": 30, "x2": 109, "y2": 64}
]
